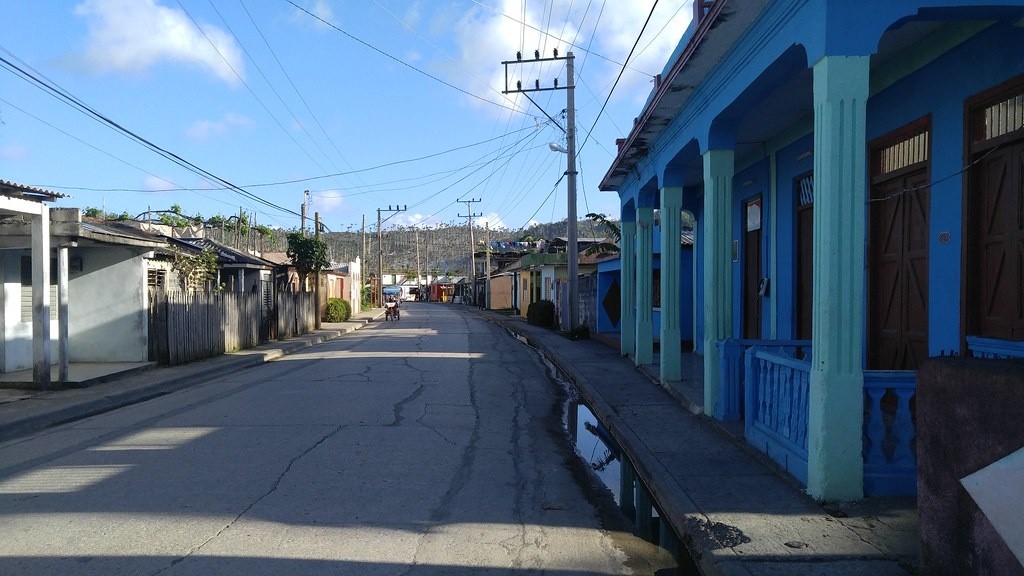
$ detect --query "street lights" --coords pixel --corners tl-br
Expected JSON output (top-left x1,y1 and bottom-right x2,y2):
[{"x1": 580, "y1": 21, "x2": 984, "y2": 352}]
[{"x1": 550, "y1": 141, "x2": 579, "y2": 330}]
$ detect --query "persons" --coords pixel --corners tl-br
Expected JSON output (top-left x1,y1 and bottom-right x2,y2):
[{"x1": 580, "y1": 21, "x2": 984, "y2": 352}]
[{"x1": 387, "y1": 295, "x2": 398, "y2": 308}]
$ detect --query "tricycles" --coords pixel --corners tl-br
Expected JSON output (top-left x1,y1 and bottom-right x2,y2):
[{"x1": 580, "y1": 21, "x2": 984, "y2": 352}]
[{"x1": 382, "y1": 285, "x2": 401, "y2": 322}]
